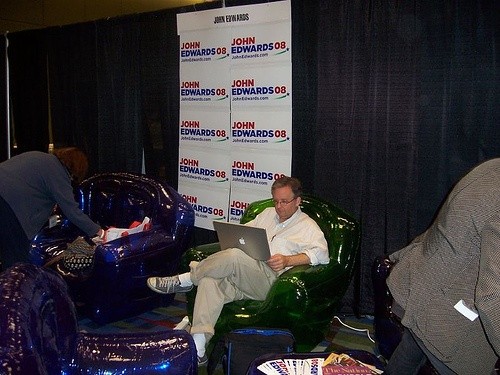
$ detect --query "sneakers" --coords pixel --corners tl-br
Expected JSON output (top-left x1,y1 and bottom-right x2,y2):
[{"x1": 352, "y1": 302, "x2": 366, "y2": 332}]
[
  {"x1": 197, "y1": 352, "x2": 208, "y2": 365},
  {"x1": 147, "y1": 273, "x2": 194, "y2": 294}
]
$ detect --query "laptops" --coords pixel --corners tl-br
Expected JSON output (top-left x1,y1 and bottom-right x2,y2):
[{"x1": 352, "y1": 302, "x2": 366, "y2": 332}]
[{"x1": 213, "y1": 221, "x2": 271, "y2": 261}]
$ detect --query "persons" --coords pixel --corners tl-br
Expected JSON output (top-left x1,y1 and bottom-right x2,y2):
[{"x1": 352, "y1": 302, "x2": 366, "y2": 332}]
[
  {"x1": 385, "y1": 159, "x2": 500, "y2": 375},
  {"x1": 147, "y1": 177, "x2": 330, "y2": 366},
  {"x1": 0, "y1": 147, "x2": 109, "y2": 272}
]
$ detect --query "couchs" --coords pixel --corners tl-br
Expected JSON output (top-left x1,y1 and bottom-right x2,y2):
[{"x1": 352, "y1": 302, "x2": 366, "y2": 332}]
[
  {"x1": 0, "y1": 261, "x2": 197, "y2": 375},
  {"x1": 28, "y1": 171, "x2": 195, "y2": 327},
  {"x1": 373, "y1": 255, "x2": 404, "y2": 361},
  {"x1": 180, "y1": 195, "x2": 362, "y2": 342}
]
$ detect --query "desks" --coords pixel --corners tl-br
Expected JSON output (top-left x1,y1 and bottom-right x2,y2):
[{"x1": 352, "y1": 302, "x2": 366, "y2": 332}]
[{"x1": 245, "y1": 350, "x2": 387, "y2": 375}]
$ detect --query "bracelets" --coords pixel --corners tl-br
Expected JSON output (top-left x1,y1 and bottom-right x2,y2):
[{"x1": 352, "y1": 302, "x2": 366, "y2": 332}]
[{"x1": 100, "y1": 229, "x2": 105, "y2": 239}]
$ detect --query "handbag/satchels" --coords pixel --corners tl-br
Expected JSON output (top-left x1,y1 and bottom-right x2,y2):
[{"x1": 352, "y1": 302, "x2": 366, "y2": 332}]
[
  {"x1": 223, "y1": 329, "x2": 294, "y2": 375},
  {"x1": 61, "y1": 237, "x2": 95, "y2": 271}
]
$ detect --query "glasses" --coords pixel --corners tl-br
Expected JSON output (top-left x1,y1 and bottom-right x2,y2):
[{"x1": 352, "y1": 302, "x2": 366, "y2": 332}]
[{"x1": 273, "y1": 198, "x2": 300, "y2": 205}]
